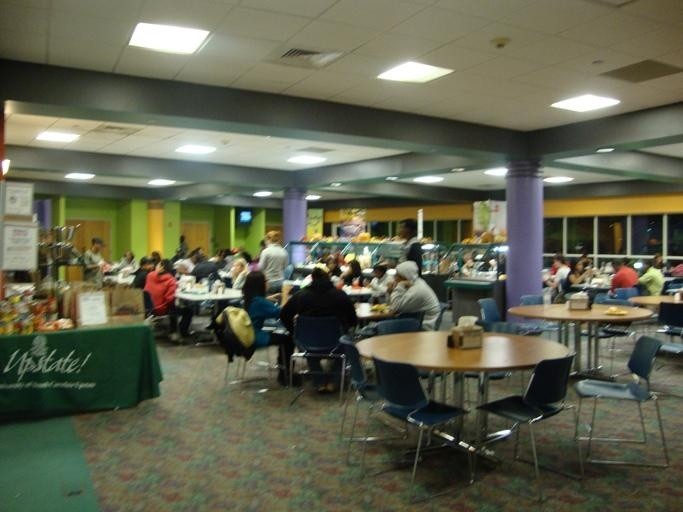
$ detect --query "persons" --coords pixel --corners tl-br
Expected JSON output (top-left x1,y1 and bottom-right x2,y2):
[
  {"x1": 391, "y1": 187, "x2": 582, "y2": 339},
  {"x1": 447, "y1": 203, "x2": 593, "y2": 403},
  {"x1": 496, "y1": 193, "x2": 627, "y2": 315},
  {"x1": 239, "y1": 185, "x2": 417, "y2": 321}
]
[
  {"x1": 143, "y1": 259, "x2": 196, "y2": 336},
  {"x1": 173, "y1": 236, "x2": 248, "y2": 290},
  {"x1": 243, "y1": 252, "x2": 258, "y2": 274},
  {"x1": 239, "y1": 270, "x2": 301, "y2": 386},
  {"x1": 114, "y1": 252, "x2": 137, "y2": 272},
  {"x1": 363, "y1": 265, "x2": 395, "y2": 302},
  {"x1": 131, "y1": 255, "x2": 155, "y2": 308},
  {"x1": 280, "y1": 264, "x2": 358, "y2": 392},
  {"x1": 83, "y1": 238, "x2": 105, "y2": 288},
  {"x1": 152, "y1": 252, "x2": 160, "y2": 266},
  {"x1": 398, "y1": 218, "x2": 425, "y2": 277},
  {"x1": 325, "y1": 256, "x2": 342, "y2": 278},
  {"x1": 543, "y1": 246, "x2": 682, "y2": 305},
  {"x1": 257, "y1": 230, "x2": 289, "y2": 296},
  {"x1": 253, "y1": 240, "x2": 266, "y2": 262},
  {"x1": 390, "y1": 260, "x2": 441, "y2": 331},
  {"x1": 343, "y1": 261, "x2": 368, "y2": 286}
]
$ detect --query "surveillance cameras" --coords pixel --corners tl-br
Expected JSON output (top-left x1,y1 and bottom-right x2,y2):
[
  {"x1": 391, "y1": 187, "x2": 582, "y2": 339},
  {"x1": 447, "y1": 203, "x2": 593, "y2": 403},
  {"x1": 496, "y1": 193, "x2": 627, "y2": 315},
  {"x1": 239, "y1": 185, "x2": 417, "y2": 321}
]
[{"x1": 488, "y1": 37, "x2": 514, "y2": 50}]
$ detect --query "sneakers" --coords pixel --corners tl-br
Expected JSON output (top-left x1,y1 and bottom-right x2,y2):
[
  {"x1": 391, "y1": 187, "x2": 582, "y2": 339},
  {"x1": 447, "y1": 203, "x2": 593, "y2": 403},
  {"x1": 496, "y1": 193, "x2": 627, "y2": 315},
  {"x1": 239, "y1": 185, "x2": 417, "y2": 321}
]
[
  {"x1": 350, "y1": 379, "x2": 364, "y2": 390},
  {"x1": 319, "y1": 383, "x2": 334, "y2": 391}
]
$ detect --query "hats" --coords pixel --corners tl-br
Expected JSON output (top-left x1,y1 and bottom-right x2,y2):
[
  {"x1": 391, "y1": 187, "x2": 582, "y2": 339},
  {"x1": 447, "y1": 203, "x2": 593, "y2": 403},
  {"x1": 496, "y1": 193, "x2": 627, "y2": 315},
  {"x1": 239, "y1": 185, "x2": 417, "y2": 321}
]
[
  {"x1": 140, "y1": 256, "x2": 156, "y2": 264},
  {"x1": 396, "y1": 260, "x2": 418, "y2": 282},
  {"x1": 93, "y1": 238, "x2": 104, "y2": 244}
]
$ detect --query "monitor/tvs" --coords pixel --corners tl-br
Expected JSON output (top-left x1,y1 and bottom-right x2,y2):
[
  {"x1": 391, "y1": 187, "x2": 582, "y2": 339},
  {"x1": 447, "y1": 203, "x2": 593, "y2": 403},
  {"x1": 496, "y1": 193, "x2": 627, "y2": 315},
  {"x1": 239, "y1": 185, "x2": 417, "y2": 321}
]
[{"x1": 239, "y1": 211, "x2": 253, "y2": 224}]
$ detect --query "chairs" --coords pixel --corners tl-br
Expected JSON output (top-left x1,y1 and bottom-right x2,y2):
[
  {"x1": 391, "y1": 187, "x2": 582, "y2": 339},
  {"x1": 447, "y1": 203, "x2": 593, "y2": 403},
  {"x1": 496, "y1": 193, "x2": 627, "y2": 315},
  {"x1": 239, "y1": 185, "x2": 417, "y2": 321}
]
[
  {"x1": 595, "y1": 300, "x2": 638, "y2": 382},
  {"x1": 465, "y1": 321, "x2": 520, "y2": 381},
  {"x1": 560, "y1": 278, "x2": 639, "y2": 301},
  {"x1": 575, "y1": 336, "x2": 672, "y2": 470},
  {"x1": 478, "y1": 298, "x2": 521, "y2": 334},
  {"x1": 290, "y1": 315, "x2": 346, "y2": 406},
  {"x1": 521, "y1": 295, "x2": 544, "y2": 305},
  {"x1": 338, "y1": 335, "x2": 380, "y2": 466},
  {"x1": 362, "y1": 356, "x2": 474, "y2": 505},
  {"x1": 376, "y1": 319, "x2": 422, "y2": 335},
  {"x1": 659, "y1": 283, "x2": 683, "y2": 356},
  {"x1": 476, "y1": 352, "x2": 585, "y2": 504}
]
